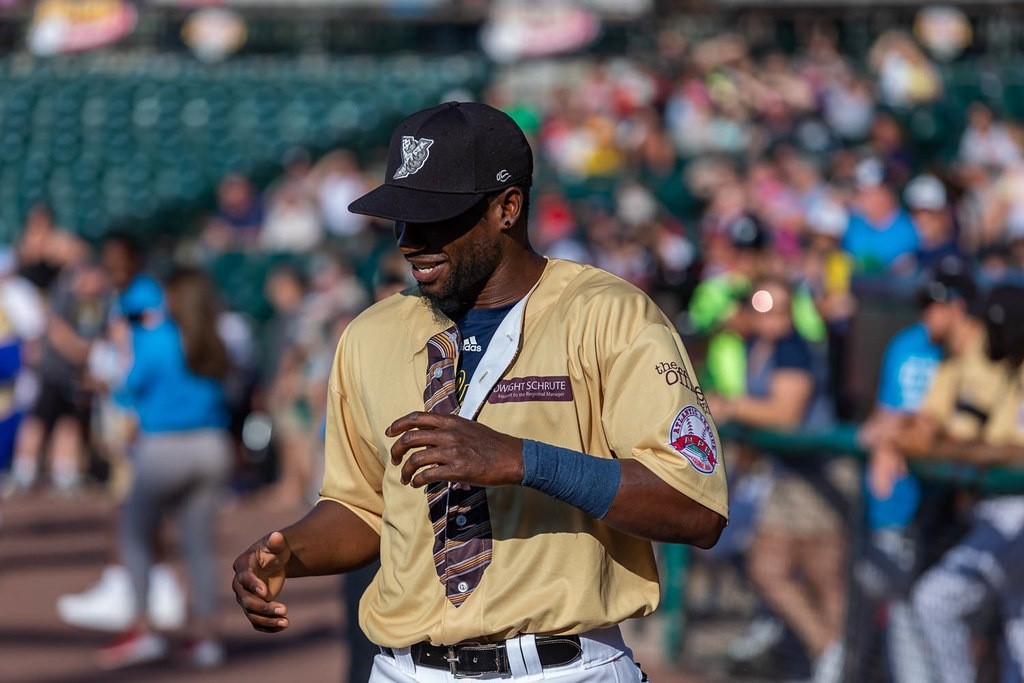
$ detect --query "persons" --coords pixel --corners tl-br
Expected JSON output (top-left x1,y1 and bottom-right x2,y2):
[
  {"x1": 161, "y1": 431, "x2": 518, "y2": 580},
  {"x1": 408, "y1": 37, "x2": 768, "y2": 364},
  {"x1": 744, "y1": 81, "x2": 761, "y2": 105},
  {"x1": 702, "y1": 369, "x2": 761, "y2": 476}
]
[
  {"x1": 97, "y1": 265, "x2": 238, "y2": 668},
  {"x1": 233, "y1": 101, "x2": 730, "y2": 683},
  {"x1": 1, "y1": 0, "x2": 1024, "y2": 682}
]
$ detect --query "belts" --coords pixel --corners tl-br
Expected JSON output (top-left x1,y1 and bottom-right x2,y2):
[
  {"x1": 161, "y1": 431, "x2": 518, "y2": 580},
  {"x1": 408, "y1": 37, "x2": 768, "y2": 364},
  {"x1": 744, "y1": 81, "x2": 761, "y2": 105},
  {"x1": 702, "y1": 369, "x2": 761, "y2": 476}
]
[{"x1": 382, "y1": 635, "x2": 581, "y2": 678}]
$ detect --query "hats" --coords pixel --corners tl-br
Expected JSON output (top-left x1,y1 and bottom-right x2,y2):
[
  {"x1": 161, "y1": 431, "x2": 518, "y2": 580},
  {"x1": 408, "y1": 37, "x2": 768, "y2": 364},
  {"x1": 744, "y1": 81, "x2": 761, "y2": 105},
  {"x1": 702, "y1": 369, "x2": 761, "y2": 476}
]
[
  {"x1": 112, "y1": 276, "x2": 166, "y2": 318},
  {"x1": 348, "y1": 102, "x2": 532, "y2": 222}
]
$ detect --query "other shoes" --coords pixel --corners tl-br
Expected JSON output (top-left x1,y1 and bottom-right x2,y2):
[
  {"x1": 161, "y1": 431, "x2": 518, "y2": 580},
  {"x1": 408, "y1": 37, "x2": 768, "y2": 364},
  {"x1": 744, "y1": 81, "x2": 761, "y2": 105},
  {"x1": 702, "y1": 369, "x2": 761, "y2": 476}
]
[
  {"x1": 59, "y1": 569, "x2": 135, "y2": 632},
  {"x1": 142, "y1": 565, "x2": 189, "y2": 630}
]
